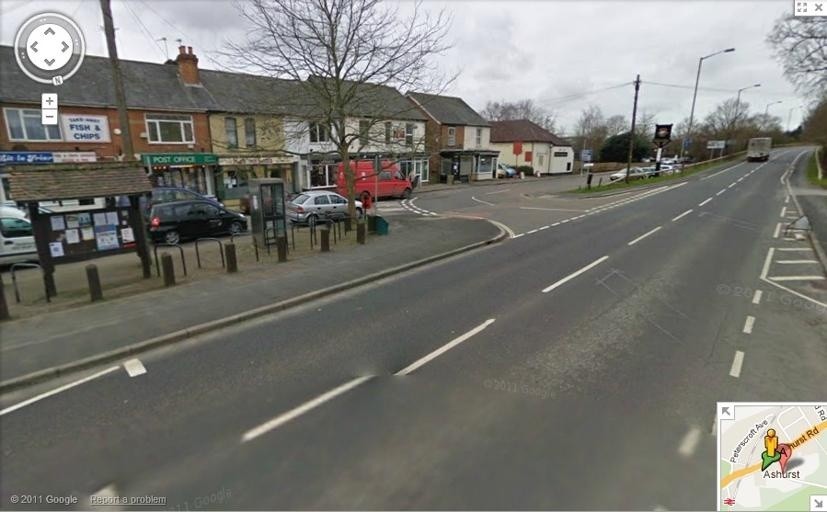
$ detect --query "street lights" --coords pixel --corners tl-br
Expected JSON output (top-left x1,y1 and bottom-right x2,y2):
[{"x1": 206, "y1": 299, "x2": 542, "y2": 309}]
[
  {"x1": 731, "y1": 84, "x2": 761, "y2": 152},
  {"x1": 766, "y1": 100, "x2": 783, "y2": 110},
  {"x1": 787, "y1": 105, "x2": 803, "y2": 130},
  {"x1": 681, "y1": 47, "x2": 736, "y2": 166}
]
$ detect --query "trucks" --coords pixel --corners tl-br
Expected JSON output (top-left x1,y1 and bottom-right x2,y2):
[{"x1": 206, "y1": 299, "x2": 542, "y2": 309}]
[{"x1": 747, "y1": 137, "x2": 772, "y2": 162}]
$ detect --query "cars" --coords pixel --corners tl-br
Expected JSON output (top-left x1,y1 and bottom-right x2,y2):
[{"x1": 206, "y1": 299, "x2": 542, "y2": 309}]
[
  {"x1": 146, "y1": 199, "x2": 247, "y2": 247},
  {"x1": 0, "y1": 200, "x2": 54, "y2": 266},
  {"x1": 117, "y1": 187, "x2": 225, "y2": 228},
  {"x1": 497, "y1": 162, "x2": 516, "y2": 177},
  {"x1": 285, "y1": 190, "x2": 363, "y2": 226},
  {"x1": 610, "y1": 156, "x2": 690, "y2": 182}
]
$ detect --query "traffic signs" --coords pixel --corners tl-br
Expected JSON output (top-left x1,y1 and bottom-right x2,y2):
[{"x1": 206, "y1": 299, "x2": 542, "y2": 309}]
[
  {"x1": 795, "y1": 0, "x2": 827, "y2": 17},
  {"x1": 582, "y1": 148, "x2": 593, "y2": 161}
]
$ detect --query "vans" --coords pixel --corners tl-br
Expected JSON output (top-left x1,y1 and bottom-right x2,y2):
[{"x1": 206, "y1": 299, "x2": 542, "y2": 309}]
[{"x1": 336, "y1": 159, "x2": 413, "y2": 201}]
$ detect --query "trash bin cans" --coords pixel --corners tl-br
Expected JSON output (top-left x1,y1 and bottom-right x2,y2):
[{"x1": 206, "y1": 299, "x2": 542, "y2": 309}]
[
  {"x1": 368, "y1": 215, "x2": 388, "y2": 235},
  {"x1": 461, "y1": 175, "x2": 468, "y2": 184}
]
